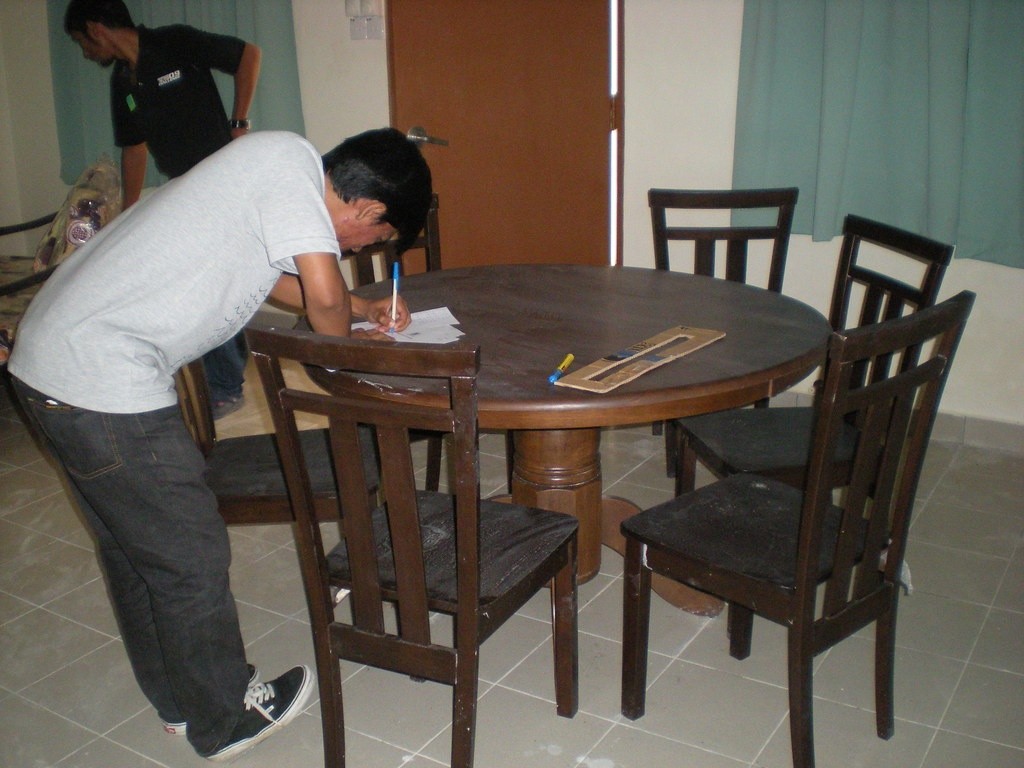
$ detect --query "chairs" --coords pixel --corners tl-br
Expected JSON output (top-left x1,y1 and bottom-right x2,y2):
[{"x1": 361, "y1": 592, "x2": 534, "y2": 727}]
[
  {"x1": 619, "y1": 186, "x2": 977, "y2": 768},
  {"x1": 171, "y1": 195, "x2": 582, "y2": 768},
  {"x1": 0, "y1": 211, "x2": 62, "y2": 443}
]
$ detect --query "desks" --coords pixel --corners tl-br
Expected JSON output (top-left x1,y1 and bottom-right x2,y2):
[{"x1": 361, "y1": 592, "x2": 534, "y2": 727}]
[{"x1": 289, "y1": 262, "x2": 832, "y2": 588}]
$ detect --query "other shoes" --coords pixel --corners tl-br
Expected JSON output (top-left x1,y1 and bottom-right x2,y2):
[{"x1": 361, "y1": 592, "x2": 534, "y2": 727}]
[{"x1": 208, "y1": 394, "x2": 245, "y2": 421}]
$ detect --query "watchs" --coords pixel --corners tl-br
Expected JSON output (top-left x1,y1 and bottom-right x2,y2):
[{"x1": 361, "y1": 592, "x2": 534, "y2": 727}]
[{"x1": 229, "y1": 120, "x2": 251, "y2": 130}]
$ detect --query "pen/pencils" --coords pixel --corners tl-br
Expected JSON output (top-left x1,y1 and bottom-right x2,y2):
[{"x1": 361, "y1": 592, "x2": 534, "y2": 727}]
[{"x1": 389, "y1": 262, "x2": 399, "y2": 332}]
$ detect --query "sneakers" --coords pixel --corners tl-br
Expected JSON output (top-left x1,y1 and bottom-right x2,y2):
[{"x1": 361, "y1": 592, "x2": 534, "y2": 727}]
[
  {"x1": 162, "y1": 664, "x2": 259, "y2": 736},
  {"x1": 204, "y1": 664, "x2": 313, "y2": 762}
]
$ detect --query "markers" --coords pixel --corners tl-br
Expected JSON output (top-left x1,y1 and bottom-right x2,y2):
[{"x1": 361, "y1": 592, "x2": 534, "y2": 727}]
[{"x1": 548, "y1": 353, "x2": 574, "y2": 383}]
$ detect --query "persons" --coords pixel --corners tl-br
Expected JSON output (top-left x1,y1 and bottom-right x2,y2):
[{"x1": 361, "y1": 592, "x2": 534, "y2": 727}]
[
  {"x1": 62, "y1": 0, "x2": 261, "y2": 419},
  {"x1": 7, "y1": 127, "x2": 433, "y2": 760}
]
